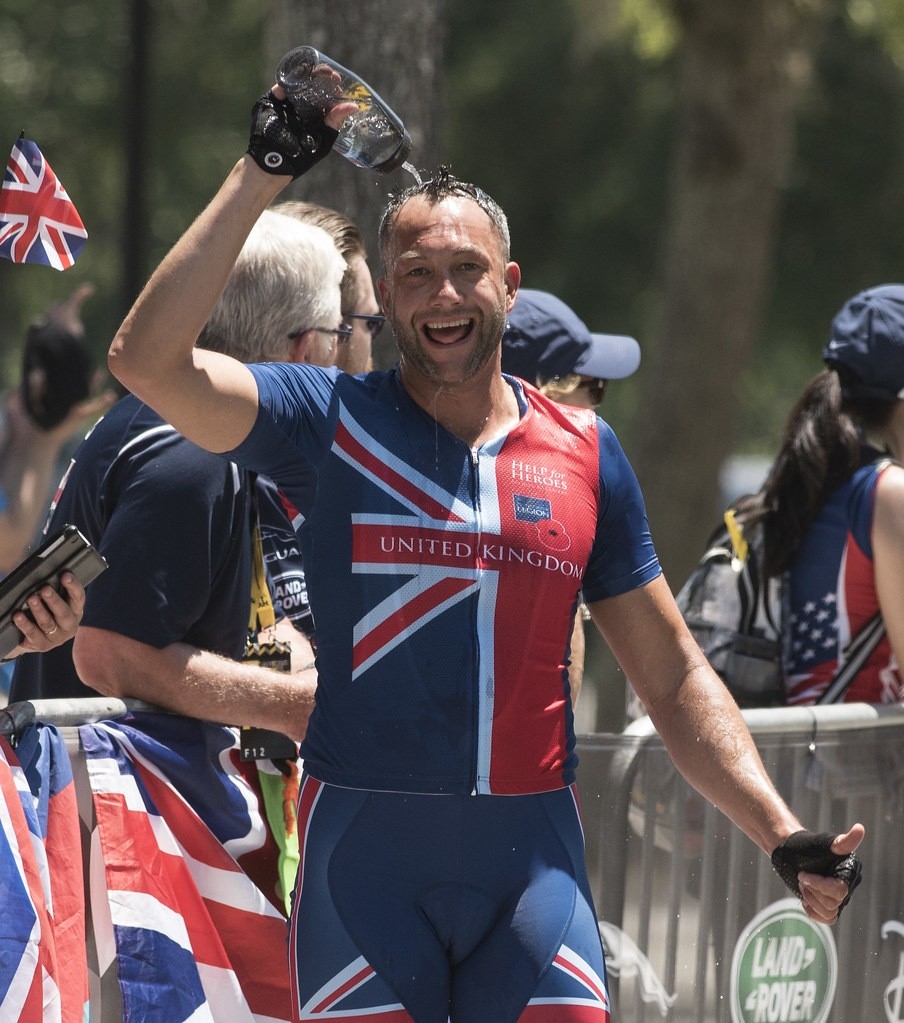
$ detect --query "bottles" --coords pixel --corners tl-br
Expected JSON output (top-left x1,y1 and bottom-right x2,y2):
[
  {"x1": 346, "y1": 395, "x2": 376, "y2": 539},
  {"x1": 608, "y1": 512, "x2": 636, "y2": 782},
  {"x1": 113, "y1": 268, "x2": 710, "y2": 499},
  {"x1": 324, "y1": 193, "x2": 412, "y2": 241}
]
[{"x1": 275, "y1": 45, "x2": 412, "y2": 174}]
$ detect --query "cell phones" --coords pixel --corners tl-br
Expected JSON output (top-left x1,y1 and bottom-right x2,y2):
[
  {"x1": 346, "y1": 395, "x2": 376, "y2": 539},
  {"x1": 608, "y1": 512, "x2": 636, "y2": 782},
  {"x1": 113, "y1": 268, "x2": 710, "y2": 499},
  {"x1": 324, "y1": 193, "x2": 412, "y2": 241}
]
[{"x1": 0, "y1": 522, "x2": 108, "y2": 662}]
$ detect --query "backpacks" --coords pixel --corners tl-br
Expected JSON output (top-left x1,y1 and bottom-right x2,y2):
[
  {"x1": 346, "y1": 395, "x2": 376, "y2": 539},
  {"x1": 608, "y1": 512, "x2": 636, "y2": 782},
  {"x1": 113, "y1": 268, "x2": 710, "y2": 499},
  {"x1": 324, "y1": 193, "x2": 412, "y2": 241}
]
[{"x1": 627, "y1": 458, "x2": 891, "y2": 737}]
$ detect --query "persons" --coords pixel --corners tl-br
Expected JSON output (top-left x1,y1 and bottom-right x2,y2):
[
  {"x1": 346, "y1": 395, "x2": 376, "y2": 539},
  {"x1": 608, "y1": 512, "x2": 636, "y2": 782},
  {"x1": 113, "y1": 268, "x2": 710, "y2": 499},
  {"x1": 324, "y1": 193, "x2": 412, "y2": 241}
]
[
  {"x1": 0, "y1": 201, "x2": 641, "y2": 1022},
  {"x1": 687, "y1": 283, "x2": 904, "y2": 1023},
  {"x1": 105, "y1": 59, "x2": 864, "y2": 1022}
]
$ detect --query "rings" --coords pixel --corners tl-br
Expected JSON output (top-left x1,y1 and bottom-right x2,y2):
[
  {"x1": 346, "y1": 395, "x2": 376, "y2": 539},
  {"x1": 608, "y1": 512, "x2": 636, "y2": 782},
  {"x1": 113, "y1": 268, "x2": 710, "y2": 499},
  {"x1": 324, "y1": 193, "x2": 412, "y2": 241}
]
[{"x1": 45, "y1": 622, "x2": 59, "y2": 636}]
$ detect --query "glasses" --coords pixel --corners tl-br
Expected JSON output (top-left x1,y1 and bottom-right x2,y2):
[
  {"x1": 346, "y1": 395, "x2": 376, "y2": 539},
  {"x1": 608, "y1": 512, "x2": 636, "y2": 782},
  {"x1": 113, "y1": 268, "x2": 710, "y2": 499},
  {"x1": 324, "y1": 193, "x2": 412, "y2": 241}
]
[
  {"x1": 343, "y1": 305, "x2": 386, "y2": 339},
  {"x1": 577, "y1": 376, "x2": 609, "y2": 406},
  {"x1": 288, "y1": 323, "x2": 353, "y2": 346}
]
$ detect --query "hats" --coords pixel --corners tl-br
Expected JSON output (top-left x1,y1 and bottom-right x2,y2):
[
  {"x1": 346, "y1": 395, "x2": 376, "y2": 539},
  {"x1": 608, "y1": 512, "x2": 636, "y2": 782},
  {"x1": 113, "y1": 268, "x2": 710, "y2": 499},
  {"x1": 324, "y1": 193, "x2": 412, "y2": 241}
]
[
  {"x1": 500, "y1": 289, "x2": 641, "y2": 391},
  {"x1": 822, "y1": 282, "x2": 904, "y2": 402}
]
